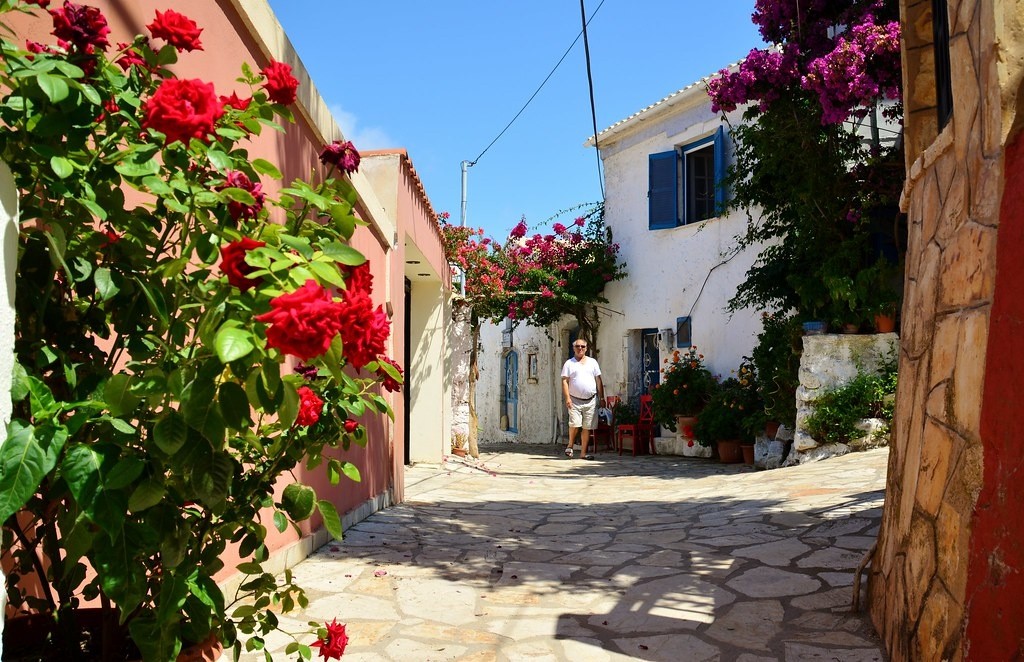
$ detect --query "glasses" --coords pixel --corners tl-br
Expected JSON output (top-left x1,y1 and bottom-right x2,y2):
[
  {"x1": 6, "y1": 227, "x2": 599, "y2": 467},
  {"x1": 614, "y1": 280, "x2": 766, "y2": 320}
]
[{"x1": 575, "y1": 345, "x2": 586, "y2": 348}]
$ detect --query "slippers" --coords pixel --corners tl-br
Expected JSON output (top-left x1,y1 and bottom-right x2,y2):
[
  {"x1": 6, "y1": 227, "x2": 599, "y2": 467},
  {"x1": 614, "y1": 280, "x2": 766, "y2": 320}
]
[
  {"x1": 565, "y1": 448, "x2": 573, "y2": 456},
  {"x1": 582, "y1": 455, "x2": 595, "y2": 460}
]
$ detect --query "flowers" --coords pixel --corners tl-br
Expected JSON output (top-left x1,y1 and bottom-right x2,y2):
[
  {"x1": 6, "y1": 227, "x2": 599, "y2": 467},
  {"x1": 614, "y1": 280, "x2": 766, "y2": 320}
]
[
  {"x1": 0, "y1": 0, "x2": 404, "y2": 662},
  {"x1": 649, "y1": 311, "x2": 803, "y2": 448}
]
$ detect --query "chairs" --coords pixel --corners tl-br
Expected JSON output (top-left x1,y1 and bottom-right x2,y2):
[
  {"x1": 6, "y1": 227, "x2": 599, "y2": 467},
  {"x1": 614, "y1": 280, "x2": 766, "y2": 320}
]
[
  {"x1": 618, "y1": 395, "x2": 656, "y2": 458},
  {"x1": 581, "y1": 396, "x2": 620, "y2": 453}
]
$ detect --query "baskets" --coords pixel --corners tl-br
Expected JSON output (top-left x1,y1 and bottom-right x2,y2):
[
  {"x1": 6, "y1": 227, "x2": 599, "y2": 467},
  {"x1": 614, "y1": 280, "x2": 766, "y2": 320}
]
[{"x1": 803, "y1": 321, "x2": 828, "y2": 335}]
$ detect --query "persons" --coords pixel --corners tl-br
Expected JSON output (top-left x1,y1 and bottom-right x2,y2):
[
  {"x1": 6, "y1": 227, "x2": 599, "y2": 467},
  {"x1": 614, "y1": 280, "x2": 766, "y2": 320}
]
[{"x1": 561, "y1": 339, "x2": 607, "y2": 460}]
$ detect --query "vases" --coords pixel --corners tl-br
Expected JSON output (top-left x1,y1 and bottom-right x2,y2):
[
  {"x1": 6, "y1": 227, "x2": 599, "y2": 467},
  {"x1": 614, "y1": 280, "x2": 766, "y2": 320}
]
[
  {"x1": 679, "y1": 416, "x2": 698, "y2": 436},
  {"x1": 659, "y1": 414, "x2": 683, "y2": 438},
  {"x1": 764, "y1": 421, "x2": 780, "y2": 440},
  {"x1": 842, "y1": 322, "x2": 861, "y2": 334},
  {"x1": 801, "y1": 322, "x2": 828, "y2": 335},
  {"x1": 0, "y1": 609, "x2": 222, "y2": 662},
  {"x1": 717, "y1": 441, "x2": 742, "y2": 464},
  {"x1": 875, "y1": 302, "x2": 900, "y2": 333},
  {"x1": 741, "y1": 446, "x2": 754, "y2": 467}
]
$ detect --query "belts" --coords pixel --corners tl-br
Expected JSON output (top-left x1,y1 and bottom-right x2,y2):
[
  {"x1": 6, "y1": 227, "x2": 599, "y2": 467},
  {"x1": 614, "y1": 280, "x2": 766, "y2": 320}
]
[{"x1": 570, "y1": 394, "x2": 595, "y2": 401}]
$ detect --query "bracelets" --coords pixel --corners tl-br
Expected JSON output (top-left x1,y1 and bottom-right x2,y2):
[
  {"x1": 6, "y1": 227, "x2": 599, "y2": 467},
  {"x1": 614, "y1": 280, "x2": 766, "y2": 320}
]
[{"x1": 599, "y1": 397, "x2": 604, "y2": 401}]
